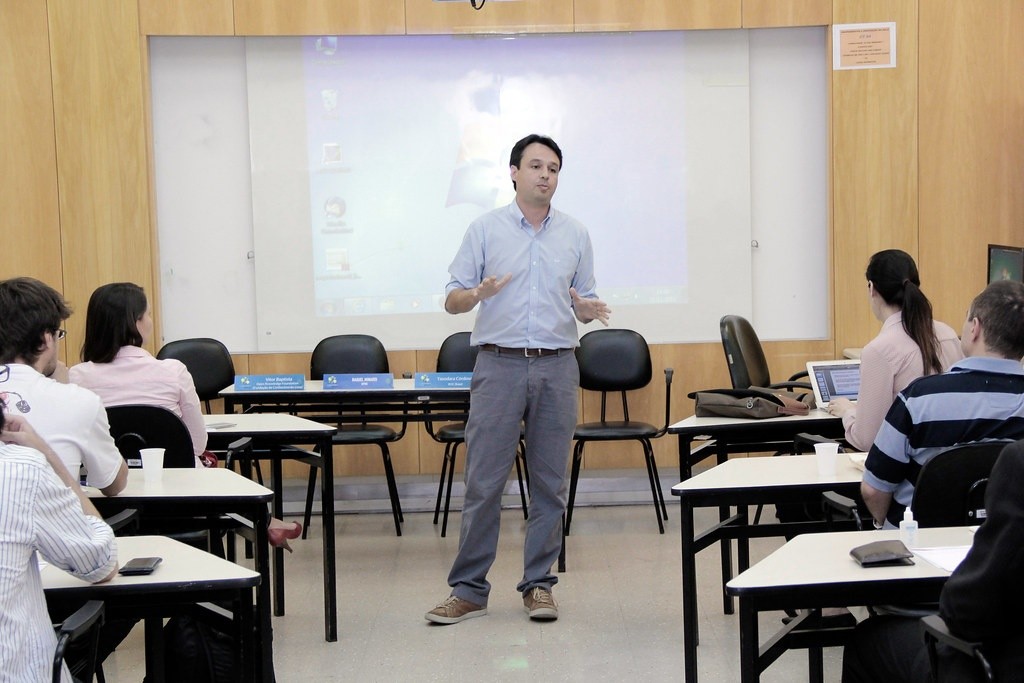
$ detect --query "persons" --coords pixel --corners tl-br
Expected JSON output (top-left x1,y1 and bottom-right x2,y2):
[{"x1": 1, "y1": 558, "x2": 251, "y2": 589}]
[
  {"x1": 0, "y1": 277, "x2": 128, "y2": 497},
  {"x1": 69, "y1": 282, "x2": 303, "y2": 554},
  {"x1": 861, "y1": 280, "x2": 1024, "y2": 528},
  {"x1": 841, "y1": 443, "x2": 1024, "y2": 683},
  {"x1": 0, "y1": 406, "x2": 119, "y2": 683},
  {"x1": 829, "y1": 249, "x2": 967, "y2": 451},
  {"x1": 424, "y1": 134, "x2": 612, "y2": 626}
]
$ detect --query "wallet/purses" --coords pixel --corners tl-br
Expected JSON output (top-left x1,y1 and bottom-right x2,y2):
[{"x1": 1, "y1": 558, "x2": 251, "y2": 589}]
[{"x1": 852, "y1": 540, "x2": 915, "y2": 567}]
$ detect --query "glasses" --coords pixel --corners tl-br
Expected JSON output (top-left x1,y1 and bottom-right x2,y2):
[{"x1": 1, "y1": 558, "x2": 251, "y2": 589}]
[
  {"x1": 0, "y1": 364, "x2": 9, "y2": 382},
  {"x1": 39, "y1": 326, "x2": 66, "y2": 342}
]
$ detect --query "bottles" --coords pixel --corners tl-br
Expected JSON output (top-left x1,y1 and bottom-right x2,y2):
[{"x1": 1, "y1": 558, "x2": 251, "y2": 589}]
[{"x1": 898, "y1": 507, "x2": 919, "y2": 548}]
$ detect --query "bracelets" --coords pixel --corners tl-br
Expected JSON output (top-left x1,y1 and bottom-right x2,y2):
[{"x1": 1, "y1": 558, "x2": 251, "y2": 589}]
[{"x1": 872, "y1": 518, "x2": 883, "y2": 528}]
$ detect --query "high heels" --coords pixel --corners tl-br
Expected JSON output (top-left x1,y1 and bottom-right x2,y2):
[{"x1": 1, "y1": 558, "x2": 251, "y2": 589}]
[{"x1": 267, "y1": 521, "x2": 301, "y2": 553}]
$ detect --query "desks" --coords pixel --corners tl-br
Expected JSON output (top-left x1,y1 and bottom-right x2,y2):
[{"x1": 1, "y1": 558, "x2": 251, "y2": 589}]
[
  {"x1": 671, "y1": 451, "x2": 890, "y2": 683},
  {"x1": 202, "y1": 413, "x2": 339, "y2": 643},
  {"x1": 667, "y1": 413, "x2": 844, "y2": 613},
  {"x1": 723, "y1": 526, "x2": 987, "y2": 683},
  {"x1": 37, "y1": 536, "x2": 265, "y2": 683},
  {"x1": 73, "y1": 465, "x2": 278, "y2": 630},
  {"x1": 218, "y1": 380, "x2": 473, "y2": 544}
]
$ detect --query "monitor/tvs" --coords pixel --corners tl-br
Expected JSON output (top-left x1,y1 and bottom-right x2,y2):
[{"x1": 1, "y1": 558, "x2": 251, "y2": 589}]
[{"x1": 987, "y1": 244, "x2": 1024, "y2": 285}]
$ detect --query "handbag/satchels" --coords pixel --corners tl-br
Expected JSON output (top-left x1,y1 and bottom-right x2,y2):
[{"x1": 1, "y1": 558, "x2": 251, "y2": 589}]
[
  {"x1": 689, "y1": 384, "x2": 811, "y2": 418},
  {"x1": 164, "y1": 614, "x2": 259, "y2": 683}
]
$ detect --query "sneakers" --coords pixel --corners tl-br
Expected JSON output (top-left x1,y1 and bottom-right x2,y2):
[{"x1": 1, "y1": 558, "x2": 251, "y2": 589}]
[
  {"x1": 526, "y1": 587, "x2": 558, "y2": 619},
  {"x1": 425, "y1": 596, "x2": 488, "y2": 624}
]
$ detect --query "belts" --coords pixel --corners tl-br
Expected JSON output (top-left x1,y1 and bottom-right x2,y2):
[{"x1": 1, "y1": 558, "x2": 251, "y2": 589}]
[{"x1": 479, "y1": 344, "x2": 572, "y2": 358}]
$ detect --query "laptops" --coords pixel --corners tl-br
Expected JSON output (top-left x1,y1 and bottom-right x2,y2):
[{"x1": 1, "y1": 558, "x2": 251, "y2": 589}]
[{"x1": 807, "y1": 359, "x2": 862, "y2": 409}]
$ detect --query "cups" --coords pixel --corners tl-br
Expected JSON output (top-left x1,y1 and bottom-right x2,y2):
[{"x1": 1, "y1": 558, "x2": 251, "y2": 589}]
[
  {"x1": 813, "y1": 442, "x2": 840, "y2": 462},
  {"x1": 139, "y1": 448, "x2": 166, "y2": 479}
]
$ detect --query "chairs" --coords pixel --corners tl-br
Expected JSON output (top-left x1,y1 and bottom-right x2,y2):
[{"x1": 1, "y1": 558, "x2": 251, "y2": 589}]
[{"x1": 53, "y1": 315, "x2": 1015, "y2": 683}]
